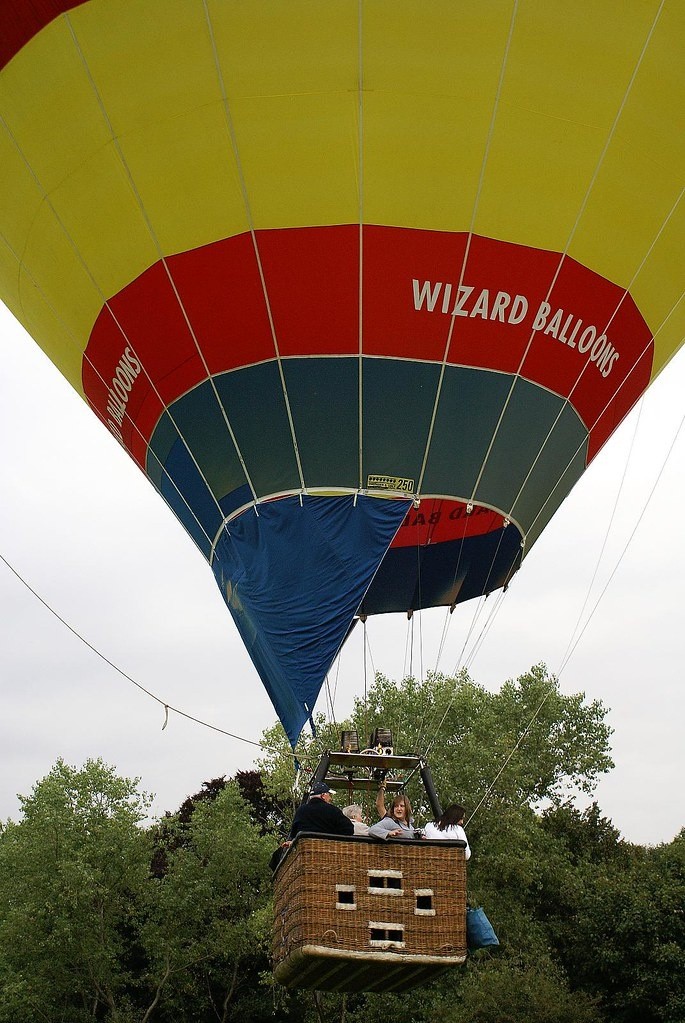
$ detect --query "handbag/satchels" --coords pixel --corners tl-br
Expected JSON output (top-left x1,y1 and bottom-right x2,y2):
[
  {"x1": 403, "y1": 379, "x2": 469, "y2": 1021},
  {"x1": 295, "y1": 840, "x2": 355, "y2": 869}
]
[{"x1": 466, "y1": 907, "x2": 500, "y2": 950}]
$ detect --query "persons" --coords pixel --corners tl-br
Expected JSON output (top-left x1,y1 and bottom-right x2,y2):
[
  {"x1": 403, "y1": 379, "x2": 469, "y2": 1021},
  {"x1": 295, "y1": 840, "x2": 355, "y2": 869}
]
[{"x1": 267, "y1": 776, "x2": 471, "y2": 873}]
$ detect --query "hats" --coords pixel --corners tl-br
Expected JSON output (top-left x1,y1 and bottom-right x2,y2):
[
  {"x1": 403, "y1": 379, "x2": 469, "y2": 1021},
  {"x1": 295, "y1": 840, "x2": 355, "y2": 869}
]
[{"x1": 310, "y1": 781, "x2": 336, "y2": 796}]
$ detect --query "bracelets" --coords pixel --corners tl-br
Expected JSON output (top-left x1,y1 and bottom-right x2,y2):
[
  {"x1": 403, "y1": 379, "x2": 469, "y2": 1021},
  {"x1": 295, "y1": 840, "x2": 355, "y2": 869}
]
[{"x1": 378, "y1": 786, "x2": 387, "y2": 791}]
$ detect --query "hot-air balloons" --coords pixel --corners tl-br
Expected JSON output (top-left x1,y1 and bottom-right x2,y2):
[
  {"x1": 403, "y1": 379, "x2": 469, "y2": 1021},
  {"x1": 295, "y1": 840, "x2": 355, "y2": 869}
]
[{"x1": 0, "y1": 0, "x2": 685, "y2": 996}]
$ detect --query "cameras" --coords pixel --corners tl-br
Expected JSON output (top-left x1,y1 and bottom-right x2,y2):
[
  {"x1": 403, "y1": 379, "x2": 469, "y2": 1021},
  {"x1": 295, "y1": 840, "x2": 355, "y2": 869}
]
[{"x1": 414, "y1": 832, "x2": 425, "y2": 840}]
